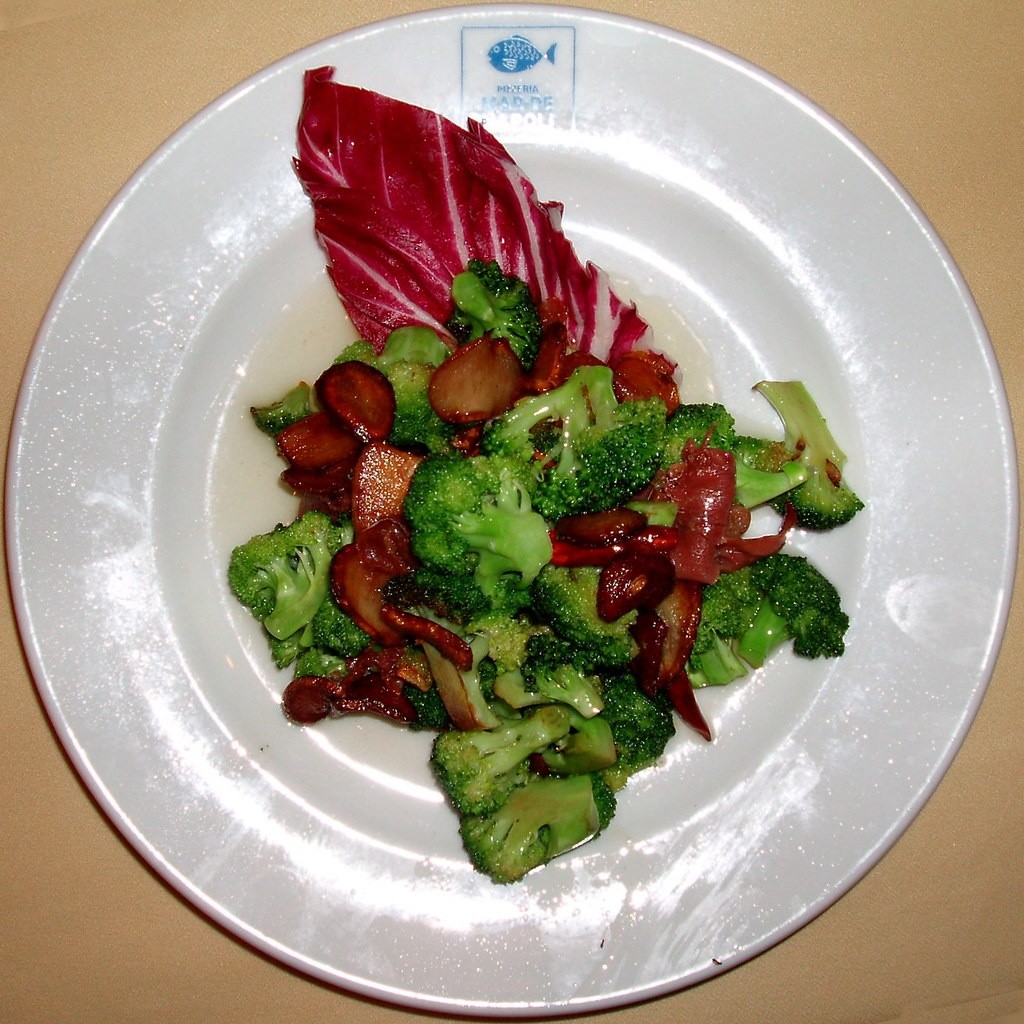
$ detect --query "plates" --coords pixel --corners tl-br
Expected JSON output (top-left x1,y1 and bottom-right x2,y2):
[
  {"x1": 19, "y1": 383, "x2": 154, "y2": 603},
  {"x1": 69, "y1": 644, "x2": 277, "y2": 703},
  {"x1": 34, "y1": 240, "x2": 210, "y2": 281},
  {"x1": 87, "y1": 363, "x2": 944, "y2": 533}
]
[{"x1": 2, "y1": 4, "x2": 1019, "y2": 1016}]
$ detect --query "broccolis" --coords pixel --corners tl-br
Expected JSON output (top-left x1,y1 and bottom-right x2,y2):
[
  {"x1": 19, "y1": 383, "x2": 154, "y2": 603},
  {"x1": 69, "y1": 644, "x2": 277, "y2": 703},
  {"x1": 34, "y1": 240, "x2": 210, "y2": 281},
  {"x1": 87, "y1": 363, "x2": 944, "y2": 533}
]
[{"x1": 222, "y1": 257, "x2": 866, "y2": 879}]
